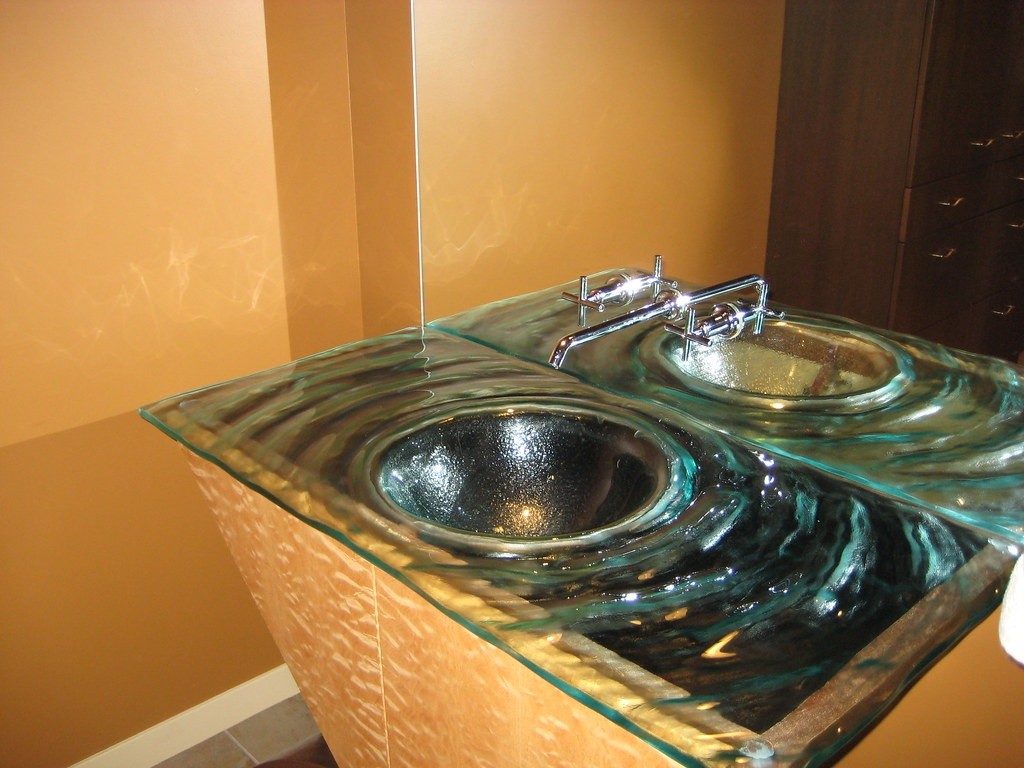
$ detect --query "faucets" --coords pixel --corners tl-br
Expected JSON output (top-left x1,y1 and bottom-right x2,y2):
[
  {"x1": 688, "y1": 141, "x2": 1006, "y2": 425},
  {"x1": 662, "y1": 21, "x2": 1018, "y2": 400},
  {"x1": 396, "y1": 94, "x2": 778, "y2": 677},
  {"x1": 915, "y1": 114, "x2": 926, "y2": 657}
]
[
  {"x1": 684, "y1": 273, "x2": 768, "y2": 335},
  {"x1": 546, "y1": 290, "x2": 684, "y2": 370}
]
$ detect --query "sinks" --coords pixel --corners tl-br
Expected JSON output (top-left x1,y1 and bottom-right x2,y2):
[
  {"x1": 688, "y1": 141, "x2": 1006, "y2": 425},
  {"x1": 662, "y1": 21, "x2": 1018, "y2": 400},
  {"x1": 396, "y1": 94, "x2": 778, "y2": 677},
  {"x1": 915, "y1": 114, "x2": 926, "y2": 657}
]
[
  {"x1": 349, "y1": 396, "x2": 697, "y2": 554},
  {"x1": 660, "y1": 317, "x2": 901, "y2": 409}
]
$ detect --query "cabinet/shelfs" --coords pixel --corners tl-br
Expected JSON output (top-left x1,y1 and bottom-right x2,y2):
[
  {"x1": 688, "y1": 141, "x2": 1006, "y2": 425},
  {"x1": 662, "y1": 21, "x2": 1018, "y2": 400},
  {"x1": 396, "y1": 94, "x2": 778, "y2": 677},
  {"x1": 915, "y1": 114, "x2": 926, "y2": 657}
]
[{"x1": 758, "y1": 0, "x2": 1024, "y2": 358}]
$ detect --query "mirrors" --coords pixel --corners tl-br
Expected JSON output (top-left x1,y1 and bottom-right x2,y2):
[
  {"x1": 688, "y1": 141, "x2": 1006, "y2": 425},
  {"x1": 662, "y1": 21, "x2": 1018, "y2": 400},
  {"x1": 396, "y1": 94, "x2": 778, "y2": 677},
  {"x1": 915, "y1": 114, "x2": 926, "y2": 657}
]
[{"x1": 403, "y1": 0, "x2": 1024, "y2": 546}]
